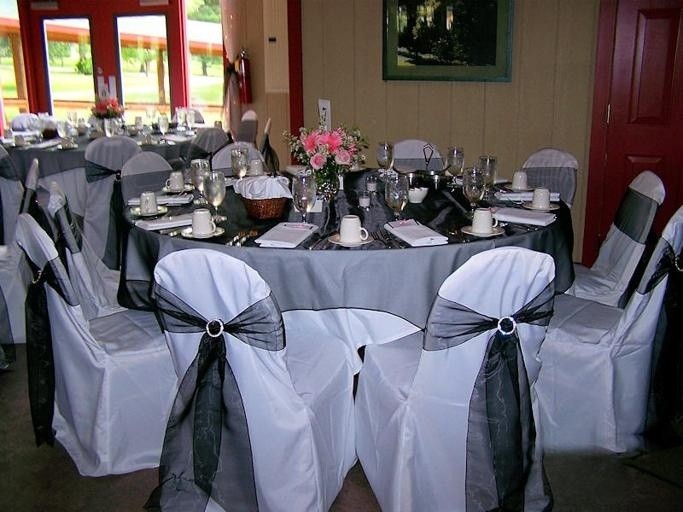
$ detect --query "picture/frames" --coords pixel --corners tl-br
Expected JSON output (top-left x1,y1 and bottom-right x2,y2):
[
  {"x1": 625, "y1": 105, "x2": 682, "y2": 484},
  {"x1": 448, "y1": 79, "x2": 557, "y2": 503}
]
[{"x1": 382, "y1": 0, "x2": 515, "y2": 84}]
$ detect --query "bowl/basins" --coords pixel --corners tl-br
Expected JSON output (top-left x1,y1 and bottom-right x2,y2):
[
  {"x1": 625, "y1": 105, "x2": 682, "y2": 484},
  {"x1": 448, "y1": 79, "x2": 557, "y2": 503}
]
[{"x1": 409, "y1": 188, "x2": 428, "y2": 203}]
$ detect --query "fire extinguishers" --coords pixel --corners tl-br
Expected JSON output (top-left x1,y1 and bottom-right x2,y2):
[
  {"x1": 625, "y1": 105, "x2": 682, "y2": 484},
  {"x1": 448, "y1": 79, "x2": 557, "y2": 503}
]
[{"x1": 234, "y1": 47, "x2": 253, "y2": 106}]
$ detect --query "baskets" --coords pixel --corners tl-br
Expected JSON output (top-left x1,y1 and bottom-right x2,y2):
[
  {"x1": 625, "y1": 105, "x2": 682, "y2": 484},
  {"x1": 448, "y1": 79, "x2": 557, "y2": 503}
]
[{"x1": 240, "y1": 195, "x2": 286, "y2": 220}]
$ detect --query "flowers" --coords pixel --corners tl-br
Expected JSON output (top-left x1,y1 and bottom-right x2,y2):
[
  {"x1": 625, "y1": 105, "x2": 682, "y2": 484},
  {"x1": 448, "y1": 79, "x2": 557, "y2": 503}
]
[
  {"x1": 91, "y1": 94, "x2": 127, "y2": 119},
  {"x1": 281, "y1": 106, "x2": 368, "y2": 193}
]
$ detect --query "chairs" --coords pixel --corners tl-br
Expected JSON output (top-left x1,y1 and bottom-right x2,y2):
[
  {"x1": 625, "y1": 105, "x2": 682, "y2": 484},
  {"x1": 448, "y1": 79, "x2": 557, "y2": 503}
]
[
  {"x1": 14, "y1": 213, "x2": 181, "y2": 471},
  {"x1": 363, "y1": 248, "x2": 557, "y2": 512},
  {"x1": 392, "y1": 140, "x2": 447, "y2": 175},
  {"x1": 41, "y1": 137, "x2": 137, "y2": 265},
  {"x1": 1, "y1": 141, "x2": 53, "y2": 249},
  {"x1": 556, "y1": 169, "x2": 665, "y2": 307},
  {"x1": 122, "y1": 152, "x2": 171, "y2": 203},
  {"x1": 48, "y1": 178, "x2": 155, "y2": 321},
  {"x1": 151, "y1": 249, "x2": 349, "y2": 505},
  {"x1": 0, "y1": 156, "x2": 39, "y2": 371},
  {"x1": 187, "y1": 127, "x2": 226, "y2": 166},
  {"x1": 258, "y1": 118, "x2": 272, "y2": 159},
  {"x1": 532, "y1": 206, "x2": 683, "y2": 454},
  {"x1": 520, "y1": 148, "x2": 578, "y2": 210},
  {"x1": 234, "y1": 109, "x2": 258, "y2": 145},
  {"x1": 210, "y1": 141, "x2": 267, "y2": 179}
]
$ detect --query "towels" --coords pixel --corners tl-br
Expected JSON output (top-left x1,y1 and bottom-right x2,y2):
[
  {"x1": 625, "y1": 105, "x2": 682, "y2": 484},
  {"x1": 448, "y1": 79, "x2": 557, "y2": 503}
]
[
  {"x1": 494, "y1": 192, "x2": 560, "y2": 202},
  {"x1": 453, "y1": 177, "x2": 507, "y2": 184},
  {"x1": 25, "y1": 138, "x2": 62, "y2": 150},
  {"x1": 135, "y1": 212, "x2": 194, "y2": 231},
  {"x1": 384, "y1": 218, "x2": 448, "y2": 247},
  {"x1": 488, "y1": 206, "x2": 556, "y2": 227},
  {"x1": 127, "y1": 194, "x2": 194, "y2": 204},
  {"x1": 254, "y1": 222, "x2": 319, "y2": 249}
]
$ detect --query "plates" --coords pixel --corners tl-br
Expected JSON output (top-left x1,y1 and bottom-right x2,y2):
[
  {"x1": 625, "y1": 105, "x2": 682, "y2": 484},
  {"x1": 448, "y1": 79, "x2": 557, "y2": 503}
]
[
  {"x1": 55, "y1": 143, "x2": 79, "y2": 149},
  {"x1": 129, "y1": 206, "x2": 168, "y2": 215},
  {"x1": 328, "y1": 233, "x2": 374, "y2": 246},
  {"x1": 521, "y1": 203, "x2": 560, "y2": 212},
  {"x1": 181, "y1": 227, "x2": 224, "y2": 238},
  {"x1": 461, "y1": 225, "x2": 504, "y2": 237},
  {"x1": 504, "y1": 184, "x2": 533, "y2": 192},
  {"x1": 162, "y1": 185, "x2": 193, "y2": 192}
]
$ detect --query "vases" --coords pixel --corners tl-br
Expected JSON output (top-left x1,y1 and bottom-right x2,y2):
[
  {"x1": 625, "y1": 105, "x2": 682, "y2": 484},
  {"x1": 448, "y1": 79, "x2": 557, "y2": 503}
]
[
  {"x1": 312, "y1": 177, "x2": 345, "y2": 235},
  {"x1": 102, "y1": 118, "x2": 118, "y2": 137}
]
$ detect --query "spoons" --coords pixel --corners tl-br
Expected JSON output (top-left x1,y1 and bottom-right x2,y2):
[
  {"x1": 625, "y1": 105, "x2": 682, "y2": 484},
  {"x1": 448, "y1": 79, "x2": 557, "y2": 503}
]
[
  {"x1": 224, "y1": 230, "x2": 245, "y2": 247},
  {"x1": 236, "y1": 229, "x2": 258, "y2": 248}
]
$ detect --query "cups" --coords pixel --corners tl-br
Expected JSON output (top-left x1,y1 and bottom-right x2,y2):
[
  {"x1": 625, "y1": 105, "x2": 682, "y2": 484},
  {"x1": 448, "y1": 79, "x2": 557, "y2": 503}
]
[
  {"x1": 359, "y1": 190, "x2": 371, "y2": 208},
  {"x1": 61, "y1": 136, "x2": 75, "y2": 147},
  {"x1": 187, "y1": 111, "x2": 194, "y2": 129},
  {"x1": 159, "y1": 114, "x2": 170, "y2": 139},
  {"x1": 15, "y1": 135, "x2": 25, "y2": 146},
  {"x1": 366, "y1": 176, "x2": 377, "y2": 191},
  {"x1": 532, "y1": 188, "x2": 549, "y2": 206},
  {"x1": 143, "y1": 133, "x2": 152, "y2": 144},
  {"x1": 165, "y1": 172, "x2": 184, "y2": 190},
  {"x1": 340, "y1": 215, "x2": 368, "y2": 242},
  {"x1": 472, "y1": 208, "x2": 499, "y2": 233},
  {"x1": 385, "y1": 175, "x2": 408, "y2": 220},
  {"x1": 251, "y1": 158, "x2": 264, "y2": 177},
  {"x1": 70, "y1": 111, "x2": 77, "y2": 123},
  {"x1": 134, "y1": 117, "x2": 143, "y2": 128},
  {"x1": 176, "y1": 108, "x2": 185, "y2": 127},
  {"x1": 190, "y1": 161, "x2": 210, "y2": 205},
  {"x1": 139, "y1": 192, "x2": 157, "y2": 213},
  {"x1": 192, "y1": 209, "x2": 215, "y2": 234},
  {"x1": 292, "y1": 169, "x2": 316, "y2": 224},
  {"x1": 202, "y1": 172, "x2": 227, "y2": 223},
  {"x1": 376, "y1": 142, "x2": 395, "y2": 177},
  {"x1": 230, "y1": 147, "x2": 248, "y2": 178},
  {"x1": 447, "y1": 145, "x2": 465, "y2": 187},
  {"x1": 465, "y1": 168, "x2": 484, "y2": 219},
  {"x1": 511, "y1": 171, "x2": 528, "y2": 190},
  {"x1": 3, "y1": 129, "x2": 12, "y2": 138}
]
